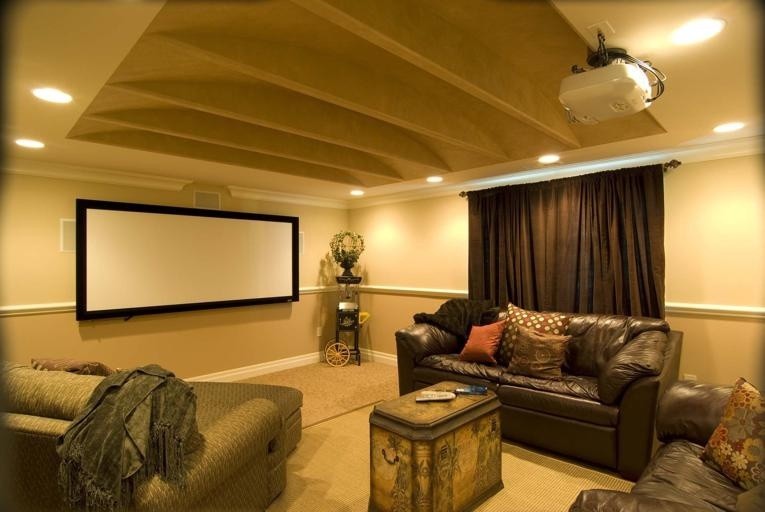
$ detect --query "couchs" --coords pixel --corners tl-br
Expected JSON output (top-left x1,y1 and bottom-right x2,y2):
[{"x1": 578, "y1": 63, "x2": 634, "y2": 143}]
[
  {"x1": 0, "y1": 362, "x2": 285, "y2": 511},
  {"x1": 393, "y1": 311, "x2": 681, "y2": 485}
]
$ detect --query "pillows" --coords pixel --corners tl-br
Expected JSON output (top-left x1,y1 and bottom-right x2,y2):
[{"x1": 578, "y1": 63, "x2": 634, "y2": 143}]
[
  {"x1": 29, "y1": 356, "x2": 114, "y2": 376},
  {"x1": 703, "y1": 377, "x2": 764, "y2": 490},
  {"x1": 457, "y1": 302, "x2": 576, "y2": 380}
]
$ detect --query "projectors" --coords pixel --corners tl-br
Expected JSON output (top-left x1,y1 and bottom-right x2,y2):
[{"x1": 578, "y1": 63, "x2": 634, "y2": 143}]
[{"x1": 559, "y1": 64, "x2": 652, "y2": 125}]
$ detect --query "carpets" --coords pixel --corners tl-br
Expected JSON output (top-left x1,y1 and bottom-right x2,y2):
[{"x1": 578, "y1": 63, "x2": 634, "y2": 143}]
[{"x1": 266, "y1": 402, "x2": 635, "y2": 510}]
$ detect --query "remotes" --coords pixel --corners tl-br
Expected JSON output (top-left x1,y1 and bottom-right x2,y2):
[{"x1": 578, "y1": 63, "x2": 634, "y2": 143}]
[{"x1": 416, "y1": 393, "x2": 455, "y2": 402}]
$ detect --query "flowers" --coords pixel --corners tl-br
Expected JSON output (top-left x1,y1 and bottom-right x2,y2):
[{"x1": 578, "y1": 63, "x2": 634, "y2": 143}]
[{"x1": 331, "y1": 230, "x2": 364, "y2": 264}]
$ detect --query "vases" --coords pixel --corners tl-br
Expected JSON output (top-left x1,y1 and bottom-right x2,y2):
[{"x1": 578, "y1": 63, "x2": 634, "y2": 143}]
[{"x1": 340, "y1": 262, "x2": 355, "y2": 276}]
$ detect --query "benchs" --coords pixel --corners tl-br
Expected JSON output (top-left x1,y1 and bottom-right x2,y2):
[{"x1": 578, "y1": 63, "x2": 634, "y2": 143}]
[{"x1": 178, "y1": 377, "x2": 304, "y2": 455}]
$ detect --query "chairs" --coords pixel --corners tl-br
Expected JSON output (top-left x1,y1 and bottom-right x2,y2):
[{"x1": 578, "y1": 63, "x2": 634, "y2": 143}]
[{"x1": 568, "y1": 380, "x2": 764, "y2": 512}]
